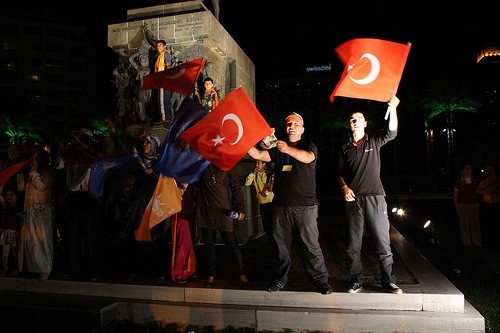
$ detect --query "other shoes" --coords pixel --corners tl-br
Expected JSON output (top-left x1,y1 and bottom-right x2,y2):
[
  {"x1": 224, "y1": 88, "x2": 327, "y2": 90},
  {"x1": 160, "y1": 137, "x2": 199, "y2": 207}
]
[
  {"x1": 240, "y1": 274, "x2": 249, "y2": 285},
  {"x1": 0, "y1": 267, "x2": 8, "y2": 276},
  {"x1": 153, "y1": 275, "x2": 170, "y2": 283},
  {"x1": 88, "y1": 270, "x2": 99, "y2": 281},
  {"x1": 176, "y1": 277, "x2": 187, "y2": 283},
  {"x1": 160, "y1": 121, "x2": 171, "y2": 130},
  {"x1": 204, "y1": 276, "x2": 216, "y2": 287},
  {"x1": 38, "y1": 272, "x2": 48, "y2": 280}
]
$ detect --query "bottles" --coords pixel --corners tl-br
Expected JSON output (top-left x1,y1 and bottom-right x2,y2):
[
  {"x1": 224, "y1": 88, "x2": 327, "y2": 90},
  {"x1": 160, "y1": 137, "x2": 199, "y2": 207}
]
[{"x1": 268, "y1": 176, "x2": 273, "y2": 186}]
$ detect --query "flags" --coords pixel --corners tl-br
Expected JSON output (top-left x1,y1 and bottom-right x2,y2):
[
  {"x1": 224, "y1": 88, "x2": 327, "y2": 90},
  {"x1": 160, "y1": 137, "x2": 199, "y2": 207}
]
[
  {"x1": 330, "y1": 38, "x2": 408, "y2": 102},
  {"x1": 142, "y1": 57, "x2": 205, "y2": 96},
  {"x1": 178, "y1": 88, "x2": 270, "y2": 171},
  {"x1": 132, "y1": 96, "x2": 211, "y2": 242}
]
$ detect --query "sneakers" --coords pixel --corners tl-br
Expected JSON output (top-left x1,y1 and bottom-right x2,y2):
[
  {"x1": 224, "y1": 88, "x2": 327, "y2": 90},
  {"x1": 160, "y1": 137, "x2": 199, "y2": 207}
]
[
  {"x1": 268, "y1": 279, "x2": 287, "y2": 292},
  {"x1": 381, "y1": 283, "x2": 404, "y2": 294},
  {"x1": 318, "y1": 282, "x2": 333, "y2": 294},
  {"x1": 347, "y1": 282, "x2": 364, "y2": 293}
]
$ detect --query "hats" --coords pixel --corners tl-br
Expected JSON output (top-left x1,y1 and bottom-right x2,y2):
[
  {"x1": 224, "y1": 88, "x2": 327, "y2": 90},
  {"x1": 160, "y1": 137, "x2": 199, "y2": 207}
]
[
  {"x1": 285, "y1": 111, "x2": 302, "y2": 123},
  {"x1": 70, "y1": 127, "x2": 94, "y2": 139},
  {"x1": 141, "y1": 133, "x2": 160, "y2": 149}
]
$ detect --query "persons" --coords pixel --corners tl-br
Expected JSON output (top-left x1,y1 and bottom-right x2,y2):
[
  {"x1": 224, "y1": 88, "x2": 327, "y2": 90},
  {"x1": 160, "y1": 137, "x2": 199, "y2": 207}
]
[
  {"x1": 454, "y1": 162, "x2": 485, "y2": 249},
  {"x1": 154, "y1": 40, "x2": 172, "y2": 125},
  {"x1": 134, "y1": 54, "x2": 153, "y2": 121},
  {"x1": 0, "y1": 118, "x2": 200, "y2": 284},
  {"x1": 194, "y1": 163, "x2": 250, "y2": 288},
  {"x1": 337, "y1": 95, "x2": 403, "y2": 294},
  {"x1": 244, "y1": 159, "x2": 276, "y2": 249},
  {"x1": 112, "y1": 64, "x2": 133, "y2": 117},
  {"x1": 201, "y1": 77, "x2": 219, "y2": 110},
  {"x1": 247, "y1": 112, "x2": 333, "y2": 296},
  {"x1": 474, "y1": 164, "x2": 500, "y2": 249}
]
